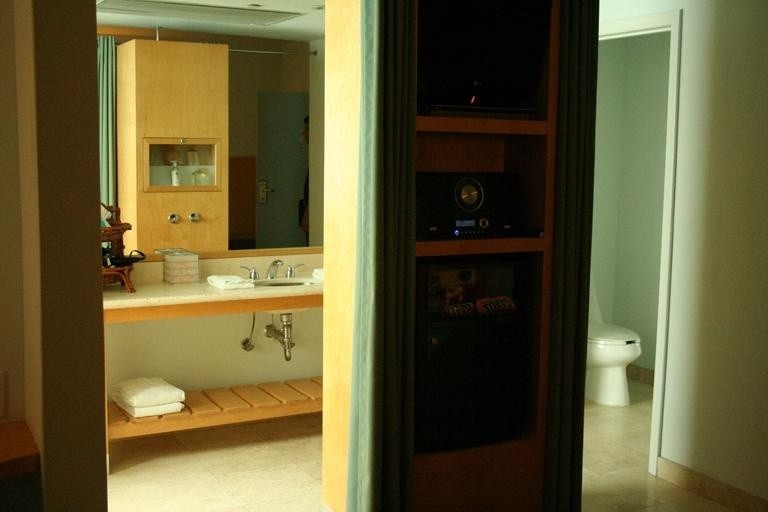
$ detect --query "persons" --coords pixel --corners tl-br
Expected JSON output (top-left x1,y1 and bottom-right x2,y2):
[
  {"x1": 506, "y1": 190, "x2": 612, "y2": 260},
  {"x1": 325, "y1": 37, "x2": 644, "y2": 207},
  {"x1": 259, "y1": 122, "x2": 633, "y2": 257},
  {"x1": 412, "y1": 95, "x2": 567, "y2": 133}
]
[{"x1": 299, "y1": 115, "x2": 309, "y2": 245}]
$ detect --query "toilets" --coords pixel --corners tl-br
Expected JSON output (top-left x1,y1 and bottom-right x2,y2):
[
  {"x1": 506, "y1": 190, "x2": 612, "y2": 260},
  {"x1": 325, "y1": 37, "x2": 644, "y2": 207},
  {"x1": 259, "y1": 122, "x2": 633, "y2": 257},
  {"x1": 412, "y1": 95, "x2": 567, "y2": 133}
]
[{"x1": 584, "y1": 278, "x2": 642, "y2": 407}]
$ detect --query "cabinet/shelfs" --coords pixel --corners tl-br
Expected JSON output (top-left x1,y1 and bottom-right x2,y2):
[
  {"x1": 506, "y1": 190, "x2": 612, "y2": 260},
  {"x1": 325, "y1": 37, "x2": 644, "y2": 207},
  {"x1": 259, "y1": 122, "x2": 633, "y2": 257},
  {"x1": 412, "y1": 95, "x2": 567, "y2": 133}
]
[
  {"x1": 141, "y1": 135, "x2": 222, "y2": 194},
  {"x1": 411, "y1": 6, "x2": 555, "y2": 512}
]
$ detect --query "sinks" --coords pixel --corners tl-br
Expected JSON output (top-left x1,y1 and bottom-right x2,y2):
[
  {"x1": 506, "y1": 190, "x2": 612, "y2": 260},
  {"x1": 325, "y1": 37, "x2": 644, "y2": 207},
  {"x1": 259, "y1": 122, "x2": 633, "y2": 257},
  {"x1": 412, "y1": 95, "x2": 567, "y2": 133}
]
[{"x1": 255, "y1": 280, "x2": 314, "y2": 287}]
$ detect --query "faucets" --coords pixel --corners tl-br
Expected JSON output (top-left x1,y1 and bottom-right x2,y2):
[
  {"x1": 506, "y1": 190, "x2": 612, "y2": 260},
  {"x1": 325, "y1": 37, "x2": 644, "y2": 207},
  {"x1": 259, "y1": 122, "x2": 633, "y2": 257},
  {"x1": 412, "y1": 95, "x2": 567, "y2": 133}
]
[{"x1": 267, "y1": 260, "x2": 283, "y2": 279}]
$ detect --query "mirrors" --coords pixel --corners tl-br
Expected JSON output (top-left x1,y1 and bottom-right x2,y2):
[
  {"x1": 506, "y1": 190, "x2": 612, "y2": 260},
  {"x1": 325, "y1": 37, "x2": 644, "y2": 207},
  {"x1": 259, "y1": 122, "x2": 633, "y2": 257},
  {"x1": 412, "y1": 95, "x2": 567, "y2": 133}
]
[{"x1": 95, "y1": 25, "x2": 327, "y2": 260}]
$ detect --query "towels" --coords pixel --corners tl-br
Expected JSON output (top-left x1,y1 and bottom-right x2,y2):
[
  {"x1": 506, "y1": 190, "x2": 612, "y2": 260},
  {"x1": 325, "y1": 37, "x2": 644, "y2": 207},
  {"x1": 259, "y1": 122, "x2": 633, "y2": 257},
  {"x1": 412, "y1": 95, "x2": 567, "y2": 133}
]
[
  {"x1": 207, "y1": 274, "x2": 253, "y2": 291},
  {"x1": 111, "y1": 375, "x2": 187, "y2": 420}
]
